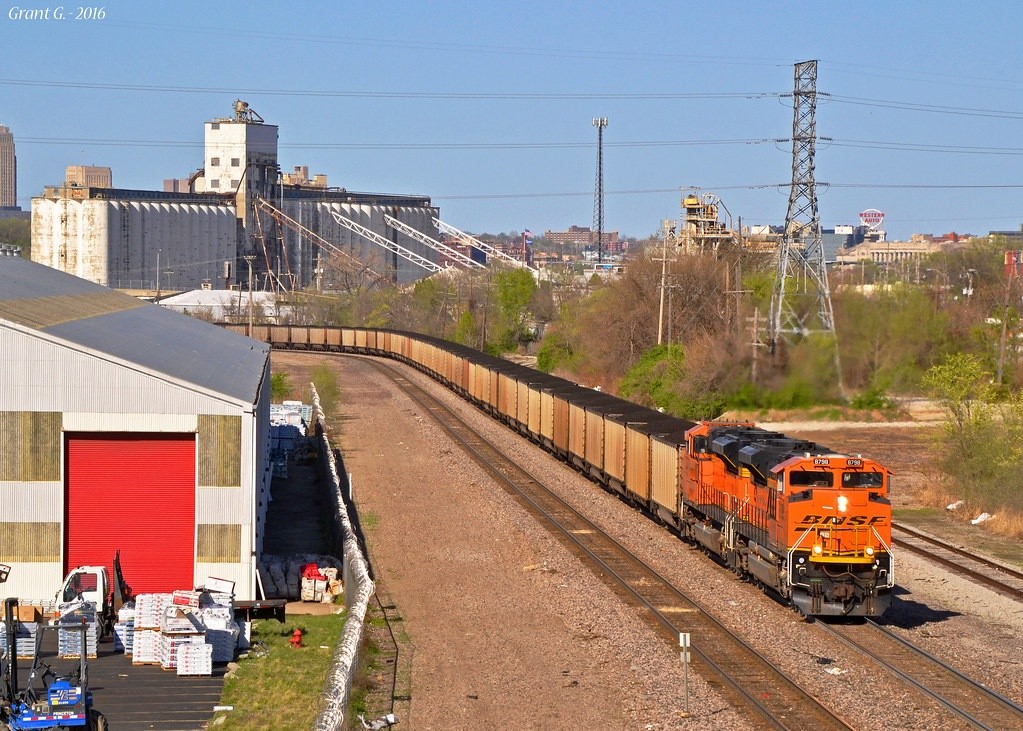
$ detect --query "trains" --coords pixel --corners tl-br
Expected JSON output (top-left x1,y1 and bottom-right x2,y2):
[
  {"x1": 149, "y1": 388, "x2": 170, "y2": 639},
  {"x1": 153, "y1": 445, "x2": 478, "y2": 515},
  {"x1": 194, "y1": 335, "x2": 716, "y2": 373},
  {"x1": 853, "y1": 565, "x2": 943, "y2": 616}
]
[{"x1": 217, "y1": 321, "x2": 894, "y2": 616}]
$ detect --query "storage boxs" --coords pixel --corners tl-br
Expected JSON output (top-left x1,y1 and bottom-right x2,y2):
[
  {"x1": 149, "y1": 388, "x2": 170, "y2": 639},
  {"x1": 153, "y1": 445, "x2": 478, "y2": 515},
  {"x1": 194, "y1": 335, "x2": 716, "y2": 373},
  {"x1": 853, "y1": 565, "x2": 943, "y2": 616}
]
[{"x1": 0, "y1": 553, "x2": 343, "y2": 731}]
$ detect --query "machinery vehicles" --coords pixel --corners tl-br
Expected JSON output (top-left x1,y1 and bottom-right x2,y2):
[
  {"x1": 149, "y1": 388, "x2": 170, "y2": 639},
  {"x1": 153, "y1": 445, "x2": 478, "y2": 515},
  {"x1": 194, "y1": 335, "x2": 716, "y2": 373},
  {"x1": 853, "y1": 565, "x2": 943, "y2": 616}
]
[{"x1": 0, "y1": 598, "x2": 109, "y2": 731}]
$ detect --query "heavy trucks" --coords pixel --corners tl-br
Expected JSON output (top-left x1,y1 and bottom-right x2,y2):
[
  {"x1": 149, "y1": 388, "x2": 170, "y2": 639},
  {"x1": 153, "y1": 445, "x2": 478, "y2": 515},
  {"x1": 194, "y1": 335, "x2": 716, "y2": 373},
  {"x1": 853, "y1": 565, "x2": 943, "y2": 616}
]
[{"x1": 48, "y1": 550, "x2": 287, "y2": 639}]
